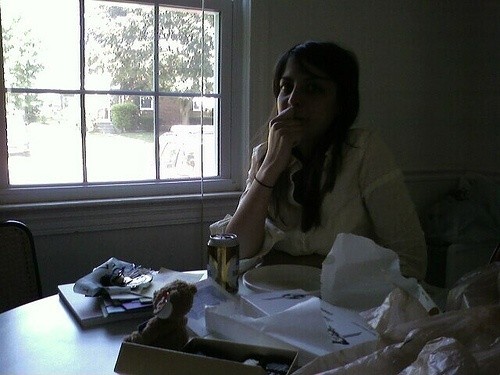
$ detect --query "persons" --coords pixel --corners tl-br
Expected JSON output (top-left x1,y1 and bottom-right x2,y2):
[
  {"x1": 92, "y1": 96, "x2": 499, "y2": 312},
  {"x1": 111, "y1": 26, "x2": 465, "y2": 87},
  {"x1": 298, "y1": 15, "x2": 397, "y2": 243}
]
[{"x1": 209, "y1": 40, "x2": 427, "y2": 283}]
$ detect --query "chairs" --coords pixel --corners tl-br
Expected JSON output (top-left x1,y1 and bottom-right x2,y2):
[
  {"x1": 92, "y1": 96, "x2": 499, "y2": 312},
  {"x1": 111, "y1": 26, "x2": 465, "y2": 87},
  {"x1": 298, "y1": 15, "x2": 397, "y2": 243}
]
[{"x1": 0, "y1": 219, "x2": 43, "y2": 314}]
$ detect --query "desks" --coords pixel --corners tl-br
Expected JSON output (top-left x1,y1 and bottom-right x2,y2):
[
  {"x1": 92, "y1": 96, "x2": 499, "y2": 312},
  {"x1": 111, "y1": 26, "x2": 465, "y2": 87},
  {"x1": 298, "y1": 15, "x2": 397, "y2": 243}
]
[{"x1": 0, "y1": 267, "x2": 443, "y2": 375}]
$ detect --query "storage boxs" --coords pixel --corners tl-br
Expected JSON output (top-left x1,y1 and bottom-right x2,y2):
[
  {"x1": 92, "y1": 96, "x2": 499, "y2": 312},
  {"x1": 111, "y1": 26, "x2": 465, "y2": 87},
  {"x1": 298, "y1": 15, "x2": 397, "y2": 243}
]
[{"x1": 114, "y1": 336, "x2": 300, "y2": 375}]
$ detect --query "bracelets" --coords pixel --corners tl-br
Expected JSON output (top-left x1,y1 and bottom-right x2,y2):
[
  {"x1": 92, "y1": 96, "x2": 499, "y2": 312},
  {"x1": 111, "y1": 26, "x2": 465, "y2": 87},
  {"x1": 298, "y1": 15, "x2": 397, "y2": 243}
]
[{"x1": 254, "y1": 174, "x2": 275, "y2": 189}]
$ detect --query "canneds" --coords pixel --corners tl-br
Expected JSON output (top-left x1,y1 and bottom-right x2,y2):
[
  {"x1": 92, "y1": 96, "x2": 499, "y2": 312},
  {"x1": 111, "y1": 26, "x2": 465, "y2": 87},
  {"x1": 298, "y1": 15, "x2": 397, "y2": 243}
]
[{"x1": 207, "y1": 233, "x2": 240, "y2": 294}]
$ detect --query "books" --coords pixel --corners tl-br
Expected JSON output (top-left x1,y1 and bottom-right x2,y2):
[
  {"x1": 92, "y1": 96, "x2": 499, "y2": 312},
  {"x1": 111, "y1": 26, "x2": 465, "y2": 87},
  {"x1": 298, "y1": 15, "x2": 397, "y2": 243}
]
[{"x1": 57, "y1": 257, "x2": 155, "y2": 330}]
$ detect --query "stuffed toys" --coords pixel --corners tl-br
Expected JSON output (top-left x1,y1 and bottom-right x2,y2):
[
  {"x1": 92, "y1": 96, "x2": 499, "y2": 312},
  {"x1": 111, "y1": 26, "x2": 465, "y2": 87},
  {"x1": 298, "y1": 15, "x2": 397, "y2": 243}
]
[{"x1": 125, "y1": 281, "x2": 198, "y2": 350}]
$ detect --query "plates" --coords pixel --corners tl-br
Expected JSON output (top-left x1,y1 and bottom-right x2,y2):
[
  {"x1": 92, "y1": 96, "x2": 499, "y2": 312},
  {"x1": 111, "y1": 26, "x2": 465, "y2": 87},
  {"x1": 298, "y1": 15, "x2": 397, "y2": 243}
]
[
  {"x1": 242, "y1": 264, "x2": 321, "y2": 298},
  {"x1": 125, "y1": 271, "x2": 153, "y2": 287}
]
